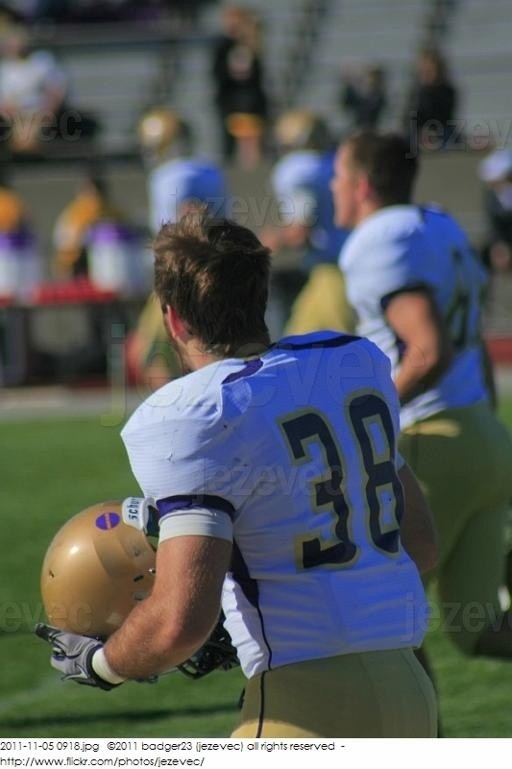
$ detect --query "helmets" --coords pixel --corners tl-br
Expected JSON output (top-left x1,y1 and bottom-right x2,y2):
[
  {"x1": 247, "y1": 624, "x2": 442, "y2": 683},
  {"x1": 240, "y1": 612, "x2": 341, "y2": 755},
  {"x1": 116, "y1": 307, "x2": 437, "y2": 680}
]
[
  {"x1": 140, "y1": 115, "x2": 190, "y2": 154},
  {"x1": 41, "y1": 494, "x2": 241, "y2": 676},
  {"x1": 271, "y1": 114, "x2": 328, "y2": 154}
]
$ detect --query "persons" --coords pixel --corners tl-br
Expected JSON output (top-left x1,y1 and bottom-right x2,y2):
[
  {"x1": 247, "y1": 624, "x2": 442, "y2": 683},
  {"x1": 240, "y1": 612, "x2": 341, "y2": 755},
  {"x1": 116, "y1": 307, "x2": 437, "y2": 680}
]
[
  {"x1": 2, "y1": 7, "x2": 512, "y2": 394},
  {"x1": 326, "y1": 120, "x2": 512, "y2": 659},
  {"x1": 32, "y1": 194, "x2": 446, "y2": 737}
]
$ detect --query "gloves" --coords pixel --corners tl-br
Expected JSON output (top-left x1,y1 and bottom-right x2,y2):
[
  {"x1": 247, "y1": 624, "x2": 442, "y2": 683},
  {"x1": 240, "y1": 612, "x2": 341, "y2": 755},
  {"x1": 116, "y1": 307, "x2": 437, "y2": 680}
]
[{"x1": 36, "y1": 622, "x2": 121, "y2": 691}]
